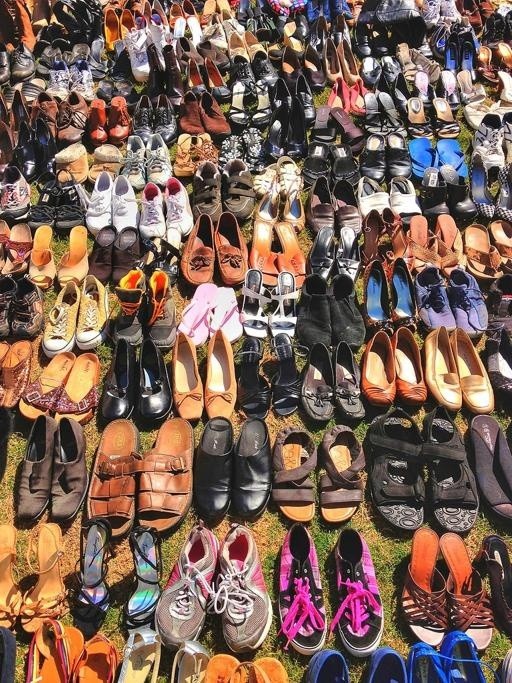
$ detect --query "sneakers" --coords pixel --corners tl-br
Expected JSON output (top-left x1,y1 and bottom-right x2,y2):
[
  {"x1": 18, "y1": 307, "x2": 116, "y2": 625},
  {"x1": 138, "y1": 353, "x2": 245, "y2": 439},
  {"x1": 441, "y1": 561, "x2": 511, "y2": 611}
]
[{"x1": 0, "y1": 0, "x2": 512, "y2": 683}]
